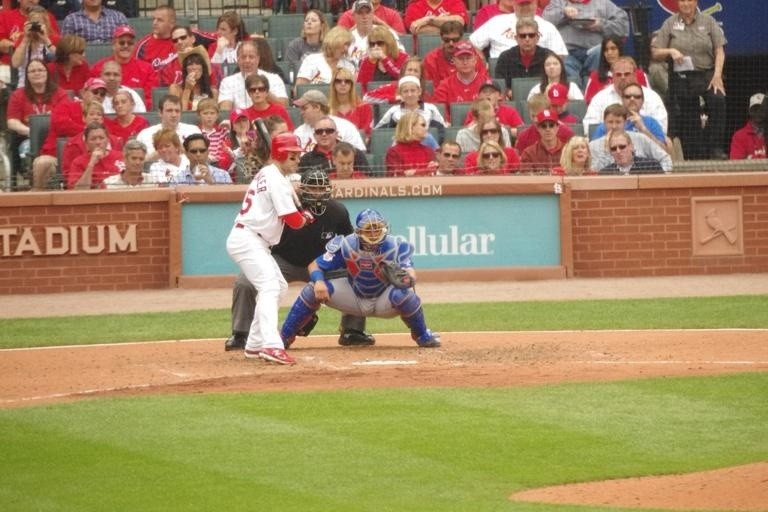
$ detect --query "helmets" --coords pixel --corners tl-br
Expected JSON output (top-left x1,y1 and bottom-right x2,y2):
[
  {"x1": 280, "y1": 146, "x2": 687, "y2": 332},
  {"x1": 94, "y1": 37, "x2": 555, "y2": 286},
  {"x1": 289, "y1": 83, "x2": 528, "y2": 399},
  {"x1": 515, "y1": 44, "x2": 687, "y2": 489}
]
[
  {"x1": 355, "y1": 207, "x2": 389, "y2": 250},
  {"x1": 298, "y1": 169, "x2": 332, "y2": 218}
]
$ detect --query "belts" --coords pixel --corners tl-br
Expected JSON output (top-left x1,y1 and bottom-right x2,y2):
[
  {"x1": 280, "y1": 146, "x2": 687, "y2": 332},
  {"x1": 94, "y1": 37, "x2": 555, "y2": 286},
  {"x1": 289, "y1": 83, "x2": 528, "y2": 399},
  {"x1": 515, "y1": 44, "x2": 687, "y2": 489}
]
[{"x1": 235, "y1": 223, "x2": 263, "y2": 238}]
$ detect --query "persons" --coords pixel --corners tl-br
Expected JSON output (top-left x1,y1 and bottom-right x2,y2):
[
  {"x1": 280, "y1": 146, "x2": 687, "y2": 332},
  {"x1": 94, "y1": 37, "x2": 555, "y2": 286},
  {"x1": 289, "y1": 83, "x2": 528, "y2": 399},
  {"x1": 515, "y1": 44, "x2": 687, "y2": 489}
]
[
  {"x1": 654, "y1": 0, "x2": 727, "y2": 160},
  {"x1": 583, "y1": 57, "x2": 669, "y2": 134},
  {"x1": 224, "y1": 167, "x2": 377, "y2": 352},
  {"x1": 553, "y1": 136, "x2": 595, "y2": 175},
  {"x1": 599, "y1": 131, "x2": 664, "y2": 174},
  {"x1": 224, "y1": 130, "x2": 317, "y2": 366},
  {"x1": 279, "y1": 207, "x2": 442, "y2": 349},
  {"x1": 286, "y1": 0, "x2": 582, "y2": 179},
  {"x1": 646, "y1": 34, "x2": 705, "y2": 106},
  {"x1": 541, "y1": 0, "x2": 630, "y2": 75},
  {"x1": 730, "y1": 93, "x2": 768, "y2": 159},
  {"x1": 588, "y1": 102, "x2": 672, "y2": 172},
  {"x1": 0, "y1": 0, "x2": 294, "y2": 192}
]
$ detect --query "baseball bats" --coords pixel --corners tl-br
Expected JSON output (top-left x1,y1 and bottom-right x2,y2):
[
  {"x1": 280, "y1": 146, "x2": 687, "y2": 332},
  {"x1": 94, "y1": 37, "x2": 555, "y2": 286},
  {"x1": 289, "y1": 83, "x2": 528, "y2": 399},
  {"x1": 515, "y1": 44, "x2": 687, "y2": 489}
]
[{"x1": 254, "y1": 118, "x2": 304, "y2": 212}]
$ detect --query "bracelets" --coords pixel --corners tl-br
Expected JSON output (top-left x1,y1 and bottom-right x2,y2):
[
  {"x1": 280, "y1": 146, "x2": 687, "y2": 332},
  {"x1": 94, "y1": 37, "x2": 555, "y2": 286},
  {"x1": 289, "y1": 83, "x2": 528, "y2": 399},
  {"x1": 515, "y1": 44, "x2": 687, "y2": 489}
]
[{"x1": 310, "y1": 270, "x2": 325, "y2": 282}]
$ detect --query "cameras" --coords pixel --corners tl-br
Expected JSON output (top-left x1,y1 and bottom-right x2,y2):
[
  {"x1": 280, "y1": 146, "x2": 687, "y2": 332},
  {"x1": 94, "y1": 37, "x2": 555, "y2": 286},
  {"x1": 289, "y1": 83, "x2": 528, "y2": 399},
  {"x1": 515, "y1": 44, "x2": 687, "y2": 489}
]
[{"x1": 31, "y1": 22, "x2": 40, "y2": 32}]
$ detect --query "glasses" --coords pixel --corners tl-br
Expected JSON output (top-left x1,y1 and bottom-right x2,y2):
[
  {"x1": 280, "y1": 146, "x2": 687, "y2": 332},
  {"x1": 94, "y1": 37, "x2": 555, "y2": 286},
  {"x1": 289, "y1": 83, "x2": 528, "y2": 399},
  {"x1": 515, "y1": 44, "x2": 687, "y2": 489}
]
[
  {"x1": 172, "y1": 34, "x2": 189, "y2": 43},
  {"x1": 91, "y1": 90, "x2": 106, "y2": 98},
  {"x1": 518, "y1": 32, "x2": 537, "y2": 39},
  {"x1": 369, "y1": 40, "x2": 386, "y2": 48},
  {"x1": 248, "y1": 86, "x2": 266, "y2": 93},
  {"x1": 27, "y1": 69, "x2": 47, "y2": 74},
  {"x1": 443, "y1": 152, "x2": 458, "y2": 158},
  {"x1": 72, "y1": 49, "x2": 85, "y2": 54},
  {"x1": 482, "y1": 128, "x2": 499, "y2": 135},
  {"x1": 335, "y1": 78, "x2": 353, "y2": 84},
  {"x1": 188, "y1": 148, "x2": 208, "y2": 154},
  {"x1": 442, "y1": 37, "x2": 461, "y2": 43},
  {"x1": 314, "y1": 128, "x2": 335, "y2": 135},
  {"x1": 118, "y1": 39, "x2": 135, "y2": 46},
  {"x1": 624, "y1": 95, "x2": 641, "y2": 100},
  {"x1": 614, "y1": 71, "x2": 632, "y2": 78},
  {"x1": 610, "y1": 144, "x2": 627, "y2": 152}
]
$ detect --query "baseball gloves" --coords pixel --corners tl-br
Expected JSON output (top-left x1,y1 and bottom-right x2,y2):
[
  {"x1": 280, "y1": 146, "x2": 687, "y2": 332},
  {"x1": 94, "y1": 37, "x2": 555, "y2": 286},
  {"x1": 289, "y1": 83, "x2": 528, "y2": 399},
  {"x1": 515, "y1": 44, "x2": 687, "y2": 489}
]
[{"x1": 385, "y1": 266, "x2": 415, "y2": 287}]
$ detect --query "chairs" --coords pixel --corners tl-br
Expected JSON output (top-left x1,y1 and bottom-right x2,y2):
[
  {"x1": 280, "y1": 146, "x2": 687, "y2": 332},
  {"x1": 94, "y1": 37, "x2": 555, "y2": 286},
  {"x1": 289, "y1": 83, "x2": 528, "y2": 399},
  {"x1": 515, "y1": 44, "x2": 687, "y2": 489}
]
[{"x1": 0, "y1": 6, "x2": 678, "y2": 190}]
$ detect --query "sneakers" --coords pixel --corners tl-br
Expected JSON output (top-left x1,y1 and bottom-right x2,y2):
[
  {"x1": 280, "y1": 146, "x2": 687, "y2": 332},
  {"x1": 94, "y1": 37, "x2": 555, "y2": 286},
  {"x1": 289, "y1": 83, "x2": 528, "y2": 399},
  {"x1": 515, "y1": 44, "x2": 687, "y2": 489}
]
[
  {"x1": 245, "y1": 348, "x2": 261, "y2": 356},
  {"x1": 225, "y1": 333, "x2": 247, "y2": 352},
  {"x1": 259, "y1": 348, "x2": 295, "y2": 365},
  {"x1": 419, "y1": 333, "x2": 441, "y2": 347},
  {"x1": 338, "y1": 329, "x2": 376, "y2": 345}
]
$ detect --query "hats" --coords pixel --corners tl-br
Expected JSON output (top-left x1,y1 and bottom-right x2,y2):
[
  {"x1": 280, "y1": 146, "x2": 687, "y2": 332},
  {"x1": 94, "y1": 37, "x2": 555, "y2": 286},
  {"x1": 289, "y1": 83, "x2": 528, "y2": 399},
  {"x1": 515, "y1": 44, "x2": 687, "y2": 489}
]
[
  {"x1": 537, "y1": 109, "x2": 559, "y2": 126},
  {"x1": 353, "y1": 1, "x2": 374, "y2": 13},
  {"x1": 84, "y1": 78, "x2": 108, "y2": 91},
  {"x1": 291, "y1": 89, "x2": 330, "y2": 106},
  {"x1": 548, "y1": 84, "x2": 567, "y2": 106},
  {"x1": 269, "y1": 133, "x2": 307, "y2": 158},
  {"x1": 750, "y1": 94, "x2": 768, "y2": 107},
  {"x1": 478, "y1": 80, "x2": 501, "y2": 97},
  {"x1": 229, "y1": 108, "x2": 248, "y2": 123},
  {"x1": 177, "y1": 44, "x2": 212, "y2": 77},
  {"x1": 113, "y1": 28, "x2": 135, "y2": 39},
  {"x1": 452, "y1": 42, "x2": 474, "y2": 57}
]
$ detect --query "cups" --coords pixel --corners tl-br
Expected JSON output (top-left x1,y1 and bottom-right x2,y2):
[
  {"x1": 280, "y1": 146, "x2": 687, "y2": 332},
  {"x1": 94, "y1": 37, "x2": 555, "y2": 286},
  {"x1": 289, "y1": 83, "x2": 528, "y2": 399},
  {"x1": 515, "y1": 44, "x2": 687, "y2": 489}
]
[{"x1": 193, "y1": 164, "x2": 206, "y2": 179}]
